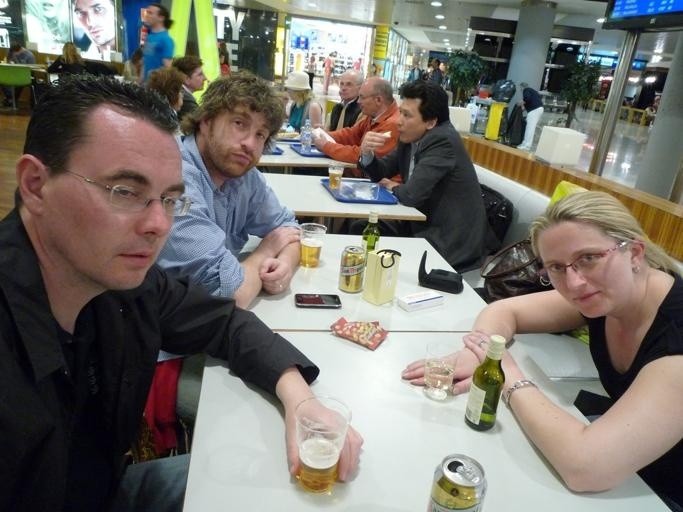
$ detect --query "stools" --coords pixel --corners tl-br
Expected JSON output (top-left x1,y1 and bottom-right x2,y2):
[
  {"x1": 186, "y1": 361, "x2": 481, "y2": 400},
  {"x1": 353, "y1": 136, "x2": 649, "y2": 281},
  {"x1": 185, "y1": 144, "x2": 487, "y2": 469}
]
[
  {"x1": 535, "y1": 126, "x2": 587, "y2": 164},
  {"x1": 447, "y1": 107, "x2": 471, "y2": 131}
]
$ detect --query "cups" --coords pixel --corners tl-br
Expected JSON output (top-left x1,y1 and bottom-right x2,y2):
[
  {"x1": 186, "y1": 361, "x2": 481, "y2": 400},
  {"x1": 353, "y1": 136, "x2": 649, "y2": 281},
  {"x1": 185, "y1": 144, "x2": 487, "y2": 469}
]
[
  {"x1": 292, "y1": 394, "x2": 348, "y2": 498},
  {"x1": 298, "y1": 222, "x2": 327, "y2": 269},
  {"x1": 326, "y1": 161, "x2": 346, "y2": 190},
  {"x1": 350, "y1": 181, "x2": 380, "y2": 201}
]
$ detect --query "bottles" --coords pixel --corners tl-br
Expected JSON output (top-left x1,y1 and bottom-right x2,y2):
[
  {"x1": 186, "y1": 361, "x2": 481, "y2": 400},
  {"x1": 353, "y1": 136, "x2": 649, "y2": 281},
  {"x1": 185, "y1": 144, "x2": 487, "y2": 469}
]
[
  {"x1": 302, "y1": 118, "x2": 312, "y2": 154},
  {"x1": 300, "y1": 125, "x2": 306, "y2": 152},
  {"x1": 464, "y1": 332, "x2": 503, "y2": 431},
  {"x1": 360, "y1": 211, "x2": 379, "y2": 269}
]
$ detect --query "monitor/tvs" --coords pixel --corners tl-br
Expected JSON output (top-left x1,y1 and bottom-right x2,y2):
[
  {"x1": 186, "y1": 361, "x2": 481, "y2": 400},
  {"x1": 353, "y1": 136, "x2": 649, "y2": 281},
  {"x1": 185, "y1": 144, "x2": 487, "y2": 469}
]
[{"x1": 601, "y1": 0, "x2": 683, "y2": 32}]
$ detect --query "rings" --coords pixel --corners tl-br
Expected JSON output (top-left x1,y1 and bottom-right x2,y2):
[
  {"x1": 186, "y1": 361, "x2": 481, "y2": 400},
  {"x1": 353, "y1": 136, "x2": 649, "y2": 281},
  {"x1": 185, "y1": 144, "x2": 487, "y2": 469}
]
[
  {"x1": 478, "y1": 339, "x2": 486, "y2": 347},
  {"x1": 277, "y1": 281, "x2": 284, "y2": 290}
]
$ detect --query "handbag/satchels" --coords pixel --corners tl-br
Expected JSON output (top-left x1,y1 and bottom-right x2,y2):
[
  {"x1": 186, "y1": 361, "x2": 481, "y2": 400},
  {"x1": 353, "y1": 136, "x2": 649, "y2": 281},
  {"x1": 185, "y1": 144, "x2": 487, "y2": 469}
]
[
  {"x1": 481, "y1": 239, "x2": 555, "y2": 300},
  {"x1": 480, "y1": 184, "x2": 513, "y2": 256}
]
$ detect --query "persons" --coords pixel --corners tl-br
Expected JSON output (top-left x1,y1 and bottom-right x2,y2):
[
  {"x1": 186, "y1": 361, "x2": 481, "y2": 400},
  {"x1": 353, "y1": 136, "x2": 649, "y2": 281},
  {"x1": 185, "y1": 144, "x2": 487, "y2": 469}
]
[
  {"x1": 216, "y1": 41, "x2": 232, "y2": 76},
  {"x1": 285, "y1": 72, "x2": 323, "y2": 136},
  {"x1": 406, "y1": 55, "x2": 450, "y2": 90},
  {"x1": 324, "y1": 70, "x2": 364, "y2": 131},
  {"x1": 1, "y1": 71, "x2": 365, "y2": 512},
  {"x1": 356, "y1": 82, "x2": 502, "y2": 274},
  {"x1": 8, "y1": 2, "x2": 207, "y2": 133},
  {"x1": 153, "y1": 68, "x2": 302, "y2": 364},
  {"x1": 516, "y1": 81, "x2": 545, "y2": 150},
  {"x1": 398, "y1": 190, "x2": 683, "y2": 507},
  {"x1": 306, "y1": 52, "x2": 338, "y2": 96},
  {"x1": 314, "y1": 76, "x2": 405, "y2": 183}
]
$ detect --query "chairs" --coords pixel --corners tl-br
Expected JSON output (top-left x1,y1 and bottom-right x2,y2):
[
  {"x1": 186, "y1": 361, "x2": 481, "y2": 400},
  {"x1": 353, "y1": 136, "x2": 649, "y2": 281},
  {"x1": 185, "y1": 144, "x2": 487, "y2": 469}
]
[{"x1": 0, "y1": 64, "x2": 32, "y2": 111}]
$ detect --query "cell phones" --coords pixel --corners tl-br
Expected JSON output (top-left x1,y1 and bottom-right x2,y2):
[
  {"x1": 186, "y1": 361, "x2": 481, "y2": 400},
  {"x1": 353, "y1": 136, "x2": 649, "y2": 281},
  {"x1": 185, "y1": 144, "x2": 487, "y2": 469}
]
[{"x1": 295, "y1": 293, "x2": 342, "y2": 308}]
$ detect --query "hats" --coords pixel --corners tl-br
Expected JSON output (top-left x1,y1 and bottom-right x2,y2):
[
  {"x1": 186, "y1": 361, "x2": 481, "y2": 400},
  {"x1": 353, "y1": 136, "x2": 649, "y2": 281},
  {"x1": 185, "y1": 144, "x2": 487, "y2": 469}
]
[{"x1": 284, "y1": 71, "x2": 311, "y2": 91}]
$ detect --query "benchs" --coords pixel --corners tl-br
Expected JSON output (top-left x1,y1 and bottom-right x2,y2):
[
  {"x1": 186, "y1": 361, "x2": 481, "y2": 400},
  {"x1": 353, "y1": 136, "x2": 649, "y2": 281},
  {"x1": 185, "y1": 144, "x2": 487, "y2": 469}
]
[{"x1": 462, "y1": 163, "x2": 683, "y2": 425}]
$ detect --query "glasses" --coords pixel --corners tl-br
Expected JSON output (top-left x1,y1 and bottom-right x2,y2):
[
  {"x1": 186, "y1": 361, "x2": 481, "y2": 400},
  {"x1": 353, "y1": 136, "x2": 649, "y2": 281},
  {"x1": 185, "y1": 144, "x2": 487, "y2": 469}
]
[
  {"x1": 47, "y1": 164, "x2": 194, "y2": 216},
  {"x1": 536, "y1": 239, "x2": 627, "y2": 282}
]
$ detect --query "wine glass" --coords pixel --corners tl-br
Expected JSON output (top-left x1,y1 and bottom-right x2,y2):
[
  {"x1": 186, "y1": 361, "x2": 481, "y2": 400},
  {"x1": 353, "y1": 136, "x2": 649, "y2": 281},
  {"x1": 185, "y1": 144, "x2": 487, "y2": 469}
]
[{"x1": 422, "y1": 342, "x2": 456, "y2": 403}]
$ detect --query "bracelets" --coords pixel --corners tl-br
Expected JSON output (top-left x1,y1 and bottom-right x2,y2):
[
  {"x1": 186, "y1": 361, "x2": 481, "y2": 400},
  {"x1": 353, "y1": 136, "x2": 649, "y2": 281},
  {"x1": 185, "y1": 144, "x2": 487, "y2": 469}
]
[{"x1": 500, "y1": 377, "x2": 540, "y2": 408}]
